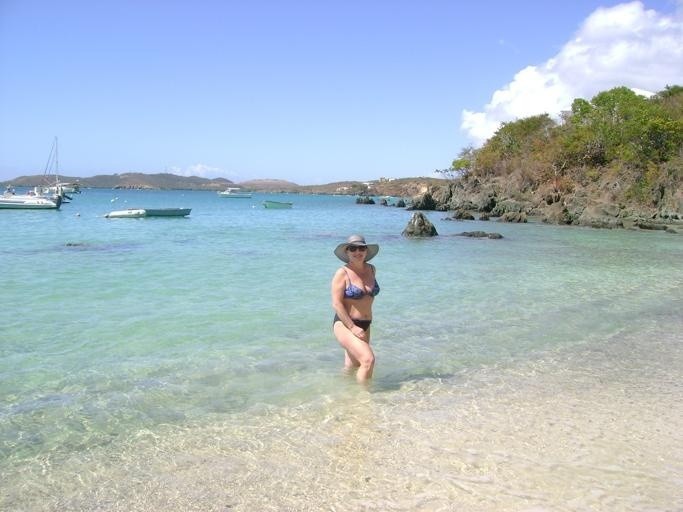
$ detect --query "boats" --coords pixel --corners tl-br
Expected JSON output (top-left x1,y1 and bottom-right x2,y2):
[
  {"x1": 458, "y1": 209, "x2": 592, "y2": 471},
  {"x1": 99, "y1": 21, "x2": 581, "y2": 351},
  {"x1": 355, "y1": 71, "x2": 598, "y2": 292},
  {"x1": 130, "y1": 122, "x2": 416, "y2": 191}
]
[
  {"x1": 0, "y1": 134, "x2": 79, "y2": 210},
  {"x1": 261, "y1": 200, "x2": 293, "y2": 209},
  {"x1": 104, "y1": 207, "x2": 192, "y2": 218},
  {"x1": 219, "y1": 186, "x2": 252, "y2": 198}
]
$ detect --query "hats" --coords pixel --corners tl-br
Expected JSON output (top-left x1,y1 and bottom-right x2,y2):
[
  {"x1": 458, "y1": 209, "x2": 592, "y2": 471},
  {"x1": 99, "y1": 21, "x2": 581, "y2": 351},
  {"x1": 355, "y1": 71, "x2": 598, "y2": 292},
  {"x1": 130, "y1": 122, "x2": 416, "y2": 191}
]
[{"x1": 333, "y1": 234, "x2": 379, "y2": 263}]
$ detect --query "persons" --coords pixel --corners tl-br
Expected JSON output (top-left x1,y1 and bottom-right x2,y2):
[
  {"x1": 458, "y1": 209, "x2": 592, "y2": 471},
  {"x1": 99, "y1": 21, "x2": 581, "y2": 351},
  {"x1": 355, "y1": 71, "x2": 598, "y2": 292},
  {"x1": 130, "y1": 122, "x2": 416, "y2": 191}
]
[{"x1": 331, "y1": 235, "x2": 380, "y2": 385}]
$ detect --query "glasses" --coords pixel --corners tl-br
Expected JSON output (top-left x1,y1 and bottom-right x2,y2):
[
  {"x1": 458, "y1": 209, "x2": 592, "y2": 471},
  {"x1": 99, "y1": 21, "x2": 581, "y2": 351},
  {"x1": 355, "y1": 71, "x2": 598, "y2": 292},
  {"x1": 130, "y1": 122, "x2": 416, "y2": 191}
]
[{"x1": 347, "y1": 246, "x2": 368, "y2": 252}]
